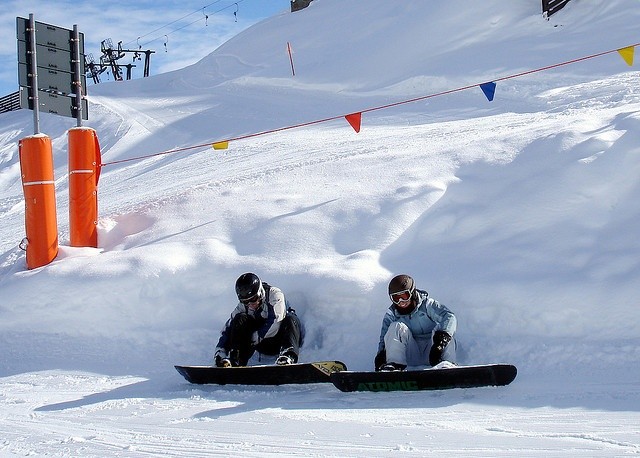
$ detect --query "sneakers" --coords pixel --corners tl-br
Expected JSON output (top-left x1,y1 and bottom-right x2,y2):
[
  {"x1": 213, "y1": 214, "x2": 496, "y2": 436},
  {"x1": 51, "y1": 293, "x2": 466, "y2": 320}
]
[
  {"x1": 379, "y1": 361, "x2": 402, "y2": 371},
  {"x1": 219, "y1": 358, "x2": 240, "y2": 367},
  {"x1": 275, "y1": 355, "x2": 294, "y2": 365},
  {"x1": 436, "y1": 359, "x2": 457, "y2": 369}
]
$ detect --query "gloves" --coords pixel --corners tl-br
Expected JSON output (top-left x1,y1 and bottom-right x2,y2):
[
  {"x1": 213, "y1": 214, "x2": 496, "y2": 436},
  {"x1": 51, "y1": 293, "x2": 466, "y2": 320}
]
[
  {"x1": 374, "y1": 351, "x2": 386, "y2": 371},
  {"x1": 214, "y1": 350, "x2": 227, "y2": 365},
  {"x1": 249, "y1": 331, "x2": 263, "y2": 347},
  {"x1": 430, "y1": 330, "x2": 452, "y2": 365}
]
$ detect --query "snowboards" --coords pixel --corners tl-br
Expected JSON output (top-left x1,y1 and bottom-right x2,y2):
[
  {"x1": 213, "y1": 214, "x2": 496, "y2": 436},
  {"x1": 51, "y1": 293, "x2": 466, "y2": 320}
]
[
  {"x1": 330, "y1": 364, "x2": 517, "y2": 392},
  {"x1": 173, "y1": 361, "x2": 347, "y2": 384}
]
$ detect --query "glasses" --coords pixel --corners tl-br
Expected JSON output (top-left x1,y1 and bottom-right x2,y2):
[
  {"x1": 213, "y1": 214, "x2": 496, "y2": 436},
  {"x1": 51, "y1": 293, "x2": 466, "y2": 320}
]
[
  {"x1": 389, "y1": 280, "x2": 415, "y2": 304},
  {"x1": 239, "y1": 282, "x2": 264, "y2": 304}
]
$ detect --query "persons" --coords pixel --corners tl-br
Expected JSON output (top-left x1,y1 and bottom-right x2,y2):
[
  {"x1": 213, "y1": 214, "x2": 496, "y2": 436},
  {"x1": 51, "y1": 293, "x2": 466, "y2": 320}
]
[
  {"x1": 373, "y1": 275, "x2": 458, "y2": 370},
  {"x1": 213, "y1": 272, "x2": 301, "y2": 367}
]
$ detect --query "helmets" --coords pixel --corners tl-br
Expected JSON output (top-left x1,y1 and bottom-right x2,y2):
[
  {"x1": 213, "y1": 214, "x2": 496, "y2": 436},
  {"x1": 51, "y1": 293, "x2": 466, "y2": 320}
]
[
  {"x1": 389, "y1": 275, "x2": 416, "y2": 299},
  {"x1": 235, "y1": 272, "x2": 260, "y2": 299}
]
